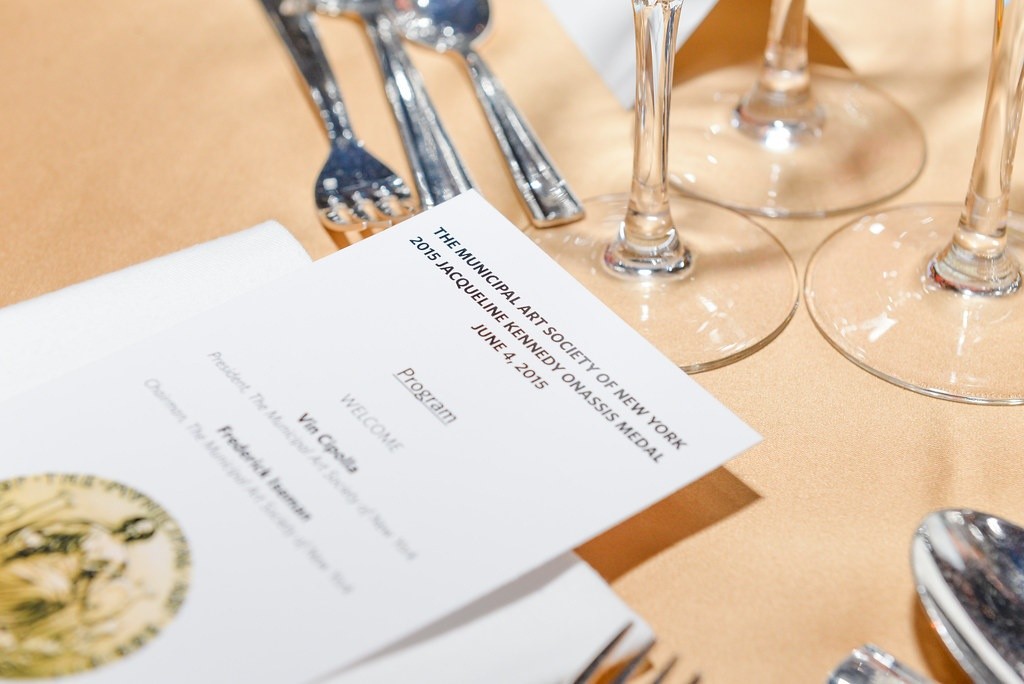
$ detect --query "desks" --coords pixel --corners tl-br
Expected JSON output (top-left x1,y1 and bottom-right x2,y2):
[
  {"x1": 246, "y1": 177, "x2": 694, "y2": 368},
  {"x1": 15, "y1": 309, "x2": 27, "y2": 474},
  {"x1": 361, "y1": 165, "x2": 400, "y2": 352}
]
[{"x1": 0, "y1": 0, "x2": 1024, "y2": 684}]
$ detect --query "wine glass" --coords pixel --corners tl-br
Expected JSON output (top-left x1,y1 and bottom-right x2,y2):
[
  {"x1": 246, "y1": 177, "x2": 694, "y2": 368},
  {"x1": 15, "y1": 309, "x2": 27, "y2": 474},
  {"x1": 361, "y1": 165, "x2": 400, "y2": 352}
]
[
  {"x1": 666, "y1": 0, "x2": 926, "y2": 217},
  {"x1": 518, "y1": 1, "x2": 800, "y2": 376},
  {"x1": 804, "y1": 0, "x2": 1024, "y2": 402}
]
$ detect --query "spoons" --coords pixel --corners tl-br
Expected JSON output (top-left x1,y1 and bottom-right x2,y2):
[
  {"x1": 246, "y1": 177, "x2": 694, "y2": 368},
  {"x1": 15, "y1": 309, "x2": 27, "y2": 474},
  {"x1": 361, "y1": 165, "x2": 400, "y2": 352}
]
[
  {"x1": 911, "y1": 508, "x2": 1024, "y2": 684},
  {"x1": 384, "y1": 0, "x2": 585, "y2": 227}
]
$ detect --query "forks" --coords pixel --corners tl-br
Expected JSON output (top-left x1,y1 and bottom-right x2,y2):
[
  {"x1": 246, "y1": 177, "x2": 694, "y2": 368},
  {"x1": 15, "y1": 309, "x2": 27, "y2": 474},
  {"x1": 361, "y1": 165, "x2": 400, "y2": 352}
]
[
  {"x1": 260, "y1": 0, "x2": 415, "y2": 233},
  {"x1": 573, "y1": 622, "x2": 703, "y2": 684}
]
